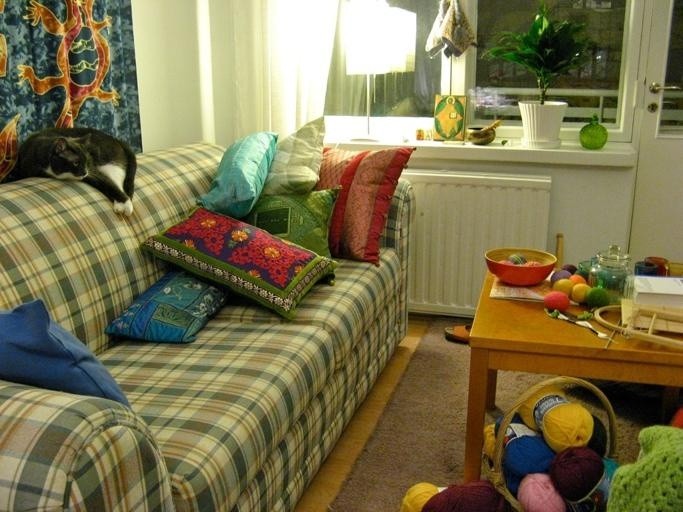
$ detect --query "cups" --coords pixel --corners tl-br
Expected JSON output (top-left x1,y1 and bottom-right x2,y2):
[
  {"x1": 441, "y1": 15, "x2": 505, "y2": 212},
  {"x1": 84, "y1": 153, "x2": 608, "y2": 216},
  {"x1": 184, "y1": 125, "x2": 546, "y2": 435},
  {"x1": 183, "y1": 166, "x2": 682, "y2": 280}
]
[
  {"x1": 635, "y1": 260, "x2": 658, "y2": 276},
  {"x1": 648, "y1": 255, "x2": 672, "y2": 277}
]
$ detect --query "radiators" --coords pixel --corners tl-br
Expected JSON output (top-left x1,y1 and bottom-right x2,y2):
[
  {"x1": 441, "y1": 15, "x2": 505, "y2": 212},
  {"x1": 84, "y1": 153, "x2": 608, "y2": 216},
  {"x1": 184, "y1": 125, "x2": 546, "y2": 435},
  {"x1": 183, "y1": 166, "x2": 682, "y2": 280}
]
[{"x1": 399, "y1": 170, "x2": 552, "y2": 318}]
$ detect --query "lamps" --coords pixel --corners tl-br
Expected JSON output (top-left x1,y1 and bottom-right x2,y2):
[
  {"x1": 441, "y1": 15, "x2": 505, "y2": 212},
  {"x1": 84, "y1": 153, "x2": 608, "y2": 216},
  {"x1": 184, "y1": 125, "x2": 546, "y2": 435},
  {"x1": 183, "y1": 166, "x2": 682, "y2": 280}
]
[{"x1": 338, "y1": 6, "x2": 420, "y2": 136}]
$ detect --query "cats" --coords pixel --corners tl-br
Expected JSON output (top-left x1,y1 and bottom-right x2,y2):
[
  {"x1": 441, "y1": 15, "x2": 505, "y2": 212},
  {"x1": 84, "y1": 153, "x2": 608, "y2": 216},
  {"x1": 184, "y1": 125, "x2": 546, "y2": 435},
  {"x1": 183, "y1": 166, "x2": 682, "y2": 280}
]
[{"x1": 0, "y1": 126, "x2": 136, "y2": 217}]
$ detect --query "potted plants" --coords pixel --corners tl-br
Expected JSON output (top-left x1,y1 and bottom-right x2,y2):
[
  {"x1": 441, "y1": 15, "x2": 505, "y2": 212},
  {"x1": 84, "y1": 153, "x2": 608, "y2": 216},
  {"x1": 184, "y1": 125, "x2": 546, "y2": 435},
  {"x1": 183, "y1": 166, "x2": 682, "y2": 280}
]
[{"x1": 480, "y1": 1, "x2": 600, "y2": 147}]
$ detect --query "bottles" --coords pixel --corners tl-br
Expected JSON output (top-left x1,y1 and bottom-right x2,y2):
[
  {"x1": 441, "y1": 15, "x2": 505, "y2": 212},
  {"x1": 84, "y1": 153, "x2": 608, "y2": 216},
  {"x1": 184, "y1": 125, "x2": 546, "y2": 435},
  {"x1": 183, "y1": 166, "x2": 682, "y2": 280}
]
[
  {"x1": 580, "y1": 112, "x2": 608, "y2": 152},
  {"x1": 588, "y1": 243, "x2": 633, "y2": 304}
]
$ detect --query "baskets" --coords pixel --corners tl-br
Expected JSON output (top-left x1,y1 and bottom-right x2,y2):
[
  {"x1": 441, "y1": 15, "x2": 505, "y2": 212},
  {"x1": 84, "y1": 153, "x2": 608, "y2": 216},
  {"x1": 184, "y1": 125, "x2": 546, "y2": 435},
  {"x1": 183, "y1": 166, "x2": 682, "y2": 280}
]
[{"x1": 482, "y1": 376, "x2": 619, "y2": 511}]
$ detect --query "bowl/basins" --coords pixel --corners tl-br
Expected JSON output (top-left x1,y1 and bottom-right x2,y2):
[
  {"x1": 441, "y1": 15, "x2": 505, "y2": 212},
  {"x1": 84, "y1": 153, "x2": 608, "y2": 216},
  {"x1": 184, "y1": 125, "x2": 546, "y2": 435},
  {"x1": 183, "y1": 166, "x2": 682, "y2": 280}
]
[
  {"x1": 484, "y1": 247, "x2": 559, "y2": 287},
  {"x1": 466, "y1": 127, "x2": 496, "y2": 145}
]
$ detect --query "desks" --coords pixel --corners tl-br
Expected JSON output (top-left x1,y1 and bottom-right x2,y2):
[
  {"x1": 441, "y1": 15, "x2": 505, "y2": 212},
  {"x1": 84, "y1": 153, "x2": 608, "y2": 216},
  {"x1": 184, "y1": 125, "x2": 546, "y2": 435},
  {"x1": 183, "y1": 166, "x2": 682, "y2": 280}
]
[{"x1": 458, "y1": 266, "x2": 683, "y2": 486}]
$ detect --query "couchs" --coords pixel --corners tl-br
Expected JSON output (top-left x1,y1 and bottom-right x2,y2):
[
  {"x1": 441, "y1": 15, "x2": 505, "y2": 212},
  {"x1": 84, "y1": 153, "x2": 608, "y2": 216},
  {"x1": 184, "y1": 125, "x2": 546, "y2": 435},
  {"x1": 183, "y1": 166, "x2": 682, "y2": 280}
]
[{"x1": 0, "y1": 139, "x2": 415, "y2": 512}]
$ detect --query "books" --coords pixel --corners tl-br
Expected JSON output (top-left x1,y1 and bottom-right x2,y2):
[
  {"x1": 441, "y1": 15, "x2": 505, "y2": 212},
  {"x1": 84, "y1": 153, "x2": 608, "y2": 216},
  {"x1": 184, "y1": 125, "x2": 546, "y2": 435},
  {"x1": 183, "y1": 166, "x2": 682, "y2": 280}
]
[{"x1": 632, "y1": 276, "x2": 683, "y2": 308}]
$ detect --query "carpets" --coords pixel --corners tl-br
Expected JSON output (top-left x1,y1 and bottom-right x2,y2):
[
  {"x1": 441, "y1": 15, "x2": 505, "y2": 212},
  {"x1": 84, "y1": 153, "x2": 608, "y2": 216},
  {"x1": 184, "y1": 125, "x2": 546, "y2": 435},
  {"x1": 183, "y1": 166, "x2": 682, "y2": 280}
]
[{"x1": 327, "y1": 313, "x2": 674, "y2": 511}]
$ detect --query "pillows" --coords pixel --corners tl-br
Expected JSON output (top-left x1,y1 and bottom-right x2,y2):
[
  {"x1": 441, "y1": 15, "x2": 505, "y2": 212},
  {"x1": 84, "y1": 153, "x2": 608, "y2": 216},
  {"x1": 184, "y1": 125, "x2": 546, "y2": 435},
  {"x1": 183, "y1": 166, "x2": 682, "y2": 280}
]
[
  {"x1": 0, "y1": 298, "x2": 135, "y2": 415},
  {"x1": 236, "y1": 183, "x2": 344, "y2": 287},
  {"x1": 138, "y1": 203, "x2": 338, "y2": 319},
  {"x1": 105, "y1": 266, "x2": 227, "y2": 343},
  {"x1": 313, "y1": 143, "x2": 415, "y2": 267},
  {"x1": 194, "y1": 132, "x2": 280, "y2": 220},
  {"x1": 264, "y1": 114, "x2": 325, "y2": 197}
]
